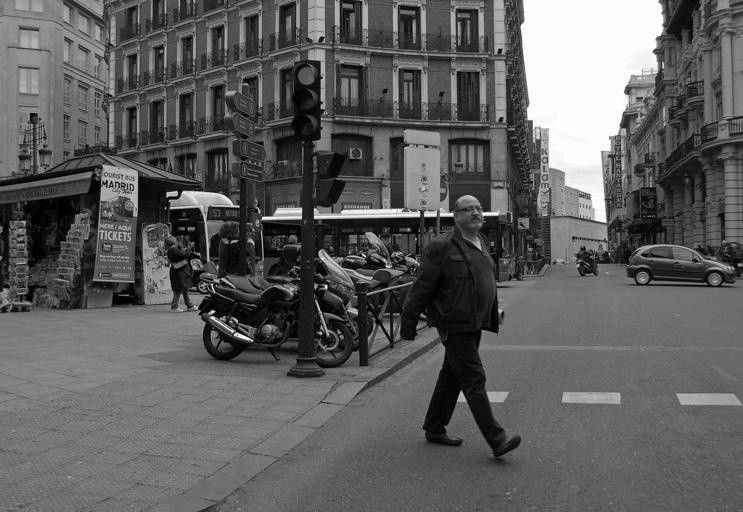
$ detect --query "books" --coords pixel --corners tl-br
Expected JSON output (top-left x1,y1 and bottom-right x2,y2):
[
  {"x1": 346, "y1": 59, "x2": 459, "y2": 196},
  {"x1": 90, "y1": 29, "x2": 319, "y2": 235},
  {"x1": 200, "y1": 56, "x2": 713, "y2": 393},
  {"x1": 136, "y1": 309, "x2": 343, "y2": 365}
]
[
  {"x1": 8, "y1": 213, "x2": 91, "y2": 308},
  {"x1": 497, "y1": 309, "x2": 505, "y2": 325}
]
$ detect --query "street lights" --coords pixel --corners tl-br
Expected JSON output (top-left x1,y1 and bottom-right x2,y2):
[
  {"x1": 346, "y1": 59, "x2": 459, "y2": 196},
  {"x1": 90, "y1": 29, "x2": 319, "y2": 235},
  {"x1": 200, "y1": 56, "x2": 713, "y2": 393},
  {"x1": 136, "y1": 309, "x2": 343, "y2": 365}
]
[{"x1": 16, "y1": 115, "x2": 52, "y2": 174}]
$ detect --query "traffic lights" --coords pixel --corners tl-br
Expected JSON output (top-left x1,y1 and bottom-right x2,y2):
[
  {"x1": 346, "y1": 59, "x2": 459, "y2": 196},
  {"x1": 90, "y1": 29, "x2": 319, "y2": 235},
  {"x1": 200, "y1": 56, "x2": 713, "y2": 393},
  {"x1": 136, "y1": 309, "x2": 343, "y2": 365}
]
[
  {"x1": 311, "y1": 150, "x2": 347, "y2": 209},
  {"x1": 288, "y1": 59, "x2": 324, "y2": 144}
]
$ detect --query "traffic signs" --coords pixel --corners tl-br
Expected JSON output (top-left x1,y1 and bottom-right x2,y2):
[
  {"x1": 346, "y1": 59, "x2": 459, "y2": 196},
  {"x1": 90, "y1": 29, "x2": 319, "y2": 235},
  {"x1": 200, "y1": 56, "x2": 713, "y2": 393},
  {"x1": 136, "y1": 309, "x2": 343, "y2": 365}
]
[
  {"x1": 231, "y1": 138, "x2": 267, "y2": 163},
  {"x1": 230, "y1": 163, "x2": 266, "y2": 185},
  {"x1": 220, "y1": 111, "x2": 257, "y2": 138},
  {"x1": 223, "y1": 90, "x2": 257, "y2": 118}
]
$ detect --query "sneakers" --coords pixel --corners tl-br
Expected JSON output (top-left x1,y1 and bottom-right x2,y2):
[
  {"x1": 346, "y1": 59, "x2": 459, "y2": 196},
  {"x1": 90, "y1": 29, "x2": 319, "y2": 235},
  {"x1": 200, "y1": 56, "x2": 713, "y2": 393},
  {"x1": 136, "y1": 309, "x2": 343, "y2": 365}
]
[
  {"x1": 493, "y1": 435, "x2": 521, "y2": 457},
  {"x1": 187, "y1": 305, "x2": 198, "y2": 311},
  {"x1": 425, "y1": 430, "x2": 463, "y2": 446},
  {"x1": 169, "y1": 307, "x2": 184, "y2": 313}
]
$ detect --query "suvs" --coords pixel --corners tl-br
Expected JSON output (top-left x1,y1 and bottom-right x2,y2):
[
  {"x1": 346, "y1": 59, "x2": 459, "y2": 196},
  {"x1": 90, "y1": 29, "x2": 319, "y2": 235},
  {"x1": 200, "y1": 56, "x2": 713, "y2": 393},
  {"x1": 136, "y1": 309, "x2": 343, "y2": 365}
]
[{"x1": 595, "y1": 250, "x2": 609, "y2": 263}]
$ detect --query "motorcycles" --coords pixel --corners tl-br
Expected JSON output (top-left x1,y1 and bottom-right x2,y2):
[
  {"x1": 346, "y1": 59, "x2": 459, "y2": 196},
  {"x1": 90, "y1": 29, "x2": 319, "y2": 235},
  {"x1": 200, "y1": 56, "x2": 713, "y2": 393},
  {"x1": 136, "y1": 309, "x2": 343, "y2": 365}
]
[
  {"x1": 507, "y1": 255, "x2": 523, "y2": 282},
  {"x1": 714, "y1": 254, "x2": 742, "y2": 277},
  {"x1": 188, "y1": 258, "x2": 210, "y2": 293},
  {"x1": 193, "y1": 263, "x2": 354, "y2": 367},
  {"x1": 266, "y1": 232, "x2": 421, "y2": 330},
  {"x1": 574, "y1": 249, "x2": 600, "y2": 278}
]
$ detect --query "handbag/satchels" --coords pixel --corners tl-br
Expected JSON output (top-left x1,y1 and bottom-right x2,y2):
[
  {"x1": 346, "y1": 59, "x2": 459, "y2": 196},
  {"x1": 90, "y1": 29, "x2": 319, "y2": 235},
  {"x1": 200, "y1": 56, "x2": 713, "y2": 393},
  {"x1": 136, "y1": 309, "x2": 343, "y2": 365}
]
[{"x1": 172, "y1": 259, "x2": 188, "y2": 270}]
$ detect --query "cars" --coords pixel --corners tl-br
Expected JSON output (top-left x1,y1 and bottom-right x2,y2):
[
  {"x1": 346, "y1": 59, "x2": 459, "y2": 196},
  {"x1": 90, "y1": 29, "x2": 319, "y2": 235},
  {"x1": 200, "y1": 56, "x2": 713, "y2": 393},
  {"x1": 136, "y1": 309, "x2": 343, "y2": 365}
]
[{"x1": 623, "y1": 243, "x2": 736, "y2": 288}]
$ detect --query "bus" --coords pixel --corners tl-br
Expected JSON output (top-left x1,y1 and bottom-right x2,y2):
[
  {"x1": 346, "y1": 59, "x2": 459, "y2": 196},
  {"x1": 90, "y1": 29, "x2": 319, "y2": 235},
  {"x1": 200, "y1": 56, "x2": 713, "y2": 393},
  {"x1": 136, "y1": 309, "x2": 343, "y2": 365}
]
[
  {"x1": 257, "y1": 205, "x2": 515, "y2": 282},
  {"x1": 161, "y1": 188, "x2": 238, "y2": 267}
]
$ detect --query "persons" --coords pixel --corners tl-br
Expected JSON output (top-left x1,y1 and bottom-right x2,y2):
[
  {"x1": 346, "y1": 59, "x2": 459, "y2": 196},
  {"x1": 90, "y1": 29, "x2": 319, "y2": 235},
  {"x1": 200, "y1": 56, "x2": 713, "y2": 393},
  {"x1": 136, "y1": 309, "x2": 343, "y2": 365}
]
[
  {"x1": 623, "y1": 246, "x2": 636, "y2": 266},
  {"x1": 165, "y1": 236, "x2": 198, "y2": 313},
  {"x1": 271, "y1": 237, "x2": 288, "y2": 249},
  {"x1": 244, "y1": 222, "x2": 257, "y2": 276},
  {"x1": 399, "y1": 194, "x2": 521, "y2": 457},
  {"x1": 217, "y1": 221, "x2": 240, "y2": 278},
  {"x1": 278, "y1": 235, "x2": 303, "y2": 275},
  {"x1": 526, "y1": 246, "x2": 544, "y2": 274},
  {"x1": 696, "y1": 240, "x2": 741, "y2": 277},
  {"x1": 578, "y1": 245, "x2": 595, "y2": 269}
]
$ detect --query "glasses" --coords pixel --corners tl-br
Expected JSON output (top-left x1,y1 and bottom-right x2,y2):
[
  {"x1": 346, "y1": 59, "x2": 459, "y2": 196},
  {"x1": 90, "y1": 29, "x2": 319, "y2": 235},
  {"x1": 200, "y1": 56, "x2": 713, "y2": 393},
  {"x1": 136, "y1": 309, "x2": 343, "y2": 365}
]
[{"x1": 455, "y1": 206, "x2": 484, "y2": 212}]
